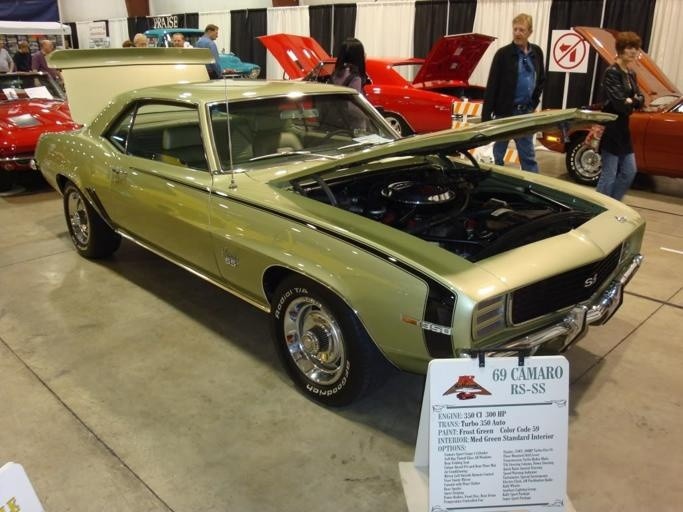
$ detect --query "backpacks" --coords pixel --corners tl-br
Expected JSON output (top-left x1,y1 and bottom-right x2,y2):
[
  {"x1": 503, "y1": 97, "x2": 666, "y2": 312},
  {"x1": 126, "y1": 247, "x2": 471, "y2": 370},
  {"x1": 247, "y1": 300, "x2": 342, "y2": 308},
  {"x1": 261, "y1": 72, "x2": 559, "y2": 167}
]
[{"x1": 320, "y1": 73, "x2": 358, "y2": 132}]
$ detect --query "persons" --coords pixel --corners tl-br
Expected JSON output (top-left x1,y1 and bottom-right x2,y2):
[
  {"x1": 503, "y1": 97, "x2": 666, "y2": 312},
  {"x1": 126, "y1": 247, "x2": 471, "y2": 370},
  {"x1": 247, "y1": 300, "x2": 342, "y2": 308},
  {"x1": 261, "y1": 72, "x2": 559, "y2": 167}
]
[
  {"x1": 12, "y1": 41, "x2": 32, "y2": 88},
  {"x1": 32, "y1": 40, "x2": 64, "y2": 94},
  {"x1": 319, "y1": 39, "x2": 367, "y2": 135},
  {"x1": 482, "y1": 14, "x2": 546, "y2": 172},
  {"x1": 194, "y1": 25, "x2": 225, "y2": 80},
  {"x1": 134, "y1": 33, "x2": 148, "y2": 48},
  {"x1": 0, "y1": 39, "x2": 14, "y2": 89},
  {"x1": 596, "y1": 32, "x2": 645, "y2": 199},
  {"x1": 172, "y1": 33, "x2": 184, "y2": 47}
]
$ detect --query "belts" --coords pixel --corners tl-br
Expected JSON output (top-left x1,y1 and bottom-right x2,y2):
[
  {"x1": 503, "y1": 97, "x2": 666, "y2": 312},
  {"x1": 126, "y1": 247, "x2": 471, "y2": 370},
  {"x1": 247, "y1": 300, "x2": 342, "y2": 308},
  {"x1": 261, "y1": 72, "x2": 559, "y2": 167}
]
[{"x1": 514, "y1": 104, "x2": 528, "y2": 110}]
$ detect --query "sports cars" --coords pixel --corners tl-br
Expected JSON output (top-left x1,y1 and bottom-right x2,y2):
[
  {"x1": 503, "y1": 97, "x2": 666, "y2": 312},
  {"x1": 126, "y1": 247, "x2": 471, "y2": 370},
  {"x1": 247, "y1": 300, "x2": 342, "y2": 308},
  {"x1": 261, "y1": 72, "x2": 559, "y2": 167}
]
[
  {"x1": 254, "y1": 33, "x2": 498, "y2": 137},
  {"x1": 35, "y1": 48, "x2": 646, "y2": 402},
  {"x1": 143, "y1": 28, "x2": 260, "y2": 80},
  {"x1": 536, "y1": 26, "x2": 683, "y2": 186},
  {"x1": 1, "y1": 72, "x2": 84, "y2": 192}
]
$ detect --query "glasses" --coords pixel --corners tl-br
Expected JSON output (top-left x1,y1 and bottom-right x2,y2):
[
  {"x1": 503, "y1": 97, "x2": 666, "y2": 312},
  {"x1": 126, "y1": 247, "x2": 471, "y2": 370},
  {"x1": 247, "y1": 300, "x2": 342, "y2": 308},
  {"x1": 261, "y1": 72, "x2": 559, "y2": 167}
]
[{"x1": 523, "y1": 58, "x2": 532, "y2": 72}]
